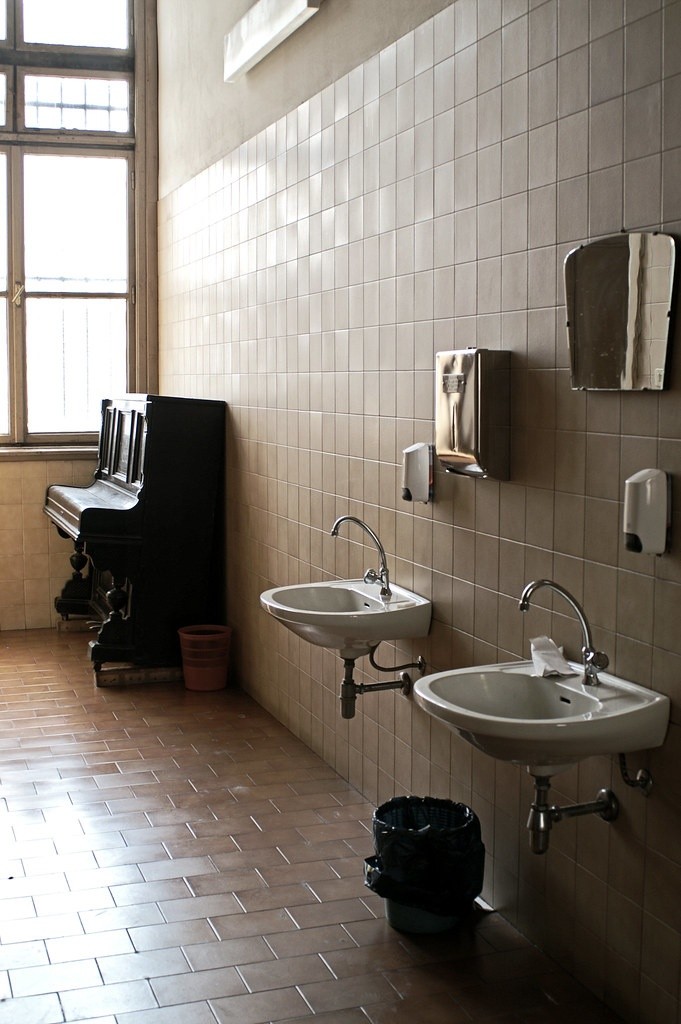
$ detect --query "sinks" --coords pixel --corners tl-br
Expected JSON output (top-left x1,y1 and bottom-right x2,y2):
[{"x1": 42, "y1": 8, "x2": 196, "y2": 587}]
[
  {"x1": 259, "y1": 577, "x2": 431, "y2": 658},
  {"x1": 412, "y1": 658, "x2": 671, "y2": 777}
]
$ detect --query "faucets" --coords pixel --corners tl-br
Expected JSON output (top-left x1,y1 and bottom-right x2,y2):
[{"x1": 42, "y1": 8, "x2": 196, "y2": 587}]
[
  {"x1": 330, "y1": 514, "x2": 392, "y2": 597},
  {"x1": 518, "y1": 579, "x2": 609, "y2": 686}
]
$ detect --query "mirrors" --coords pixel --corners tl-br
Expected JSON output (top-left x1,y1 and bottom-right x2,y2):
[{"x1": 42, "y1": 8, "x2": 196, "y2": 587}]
[{"x1": 562, "y1": 232, "x2": 676, "y2": 393}]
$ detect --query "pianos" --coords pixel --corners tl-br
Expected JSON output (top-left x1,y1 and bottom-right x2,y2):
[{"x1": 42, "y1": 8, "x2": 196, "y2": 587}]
[{"x1": 42, "y1": 390, "x2": 228, "y2": 692}]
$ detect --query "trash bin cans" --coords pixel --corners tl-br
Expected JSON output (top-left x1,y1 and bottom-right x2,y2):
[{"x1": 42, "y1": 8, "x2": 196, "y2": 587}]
[
  {"x1": 177, "y1": 623, "x2": 234, "y2": 692},
  {"x1": 363, "y1": 795, "x2": 487, "y2": 942}
]
